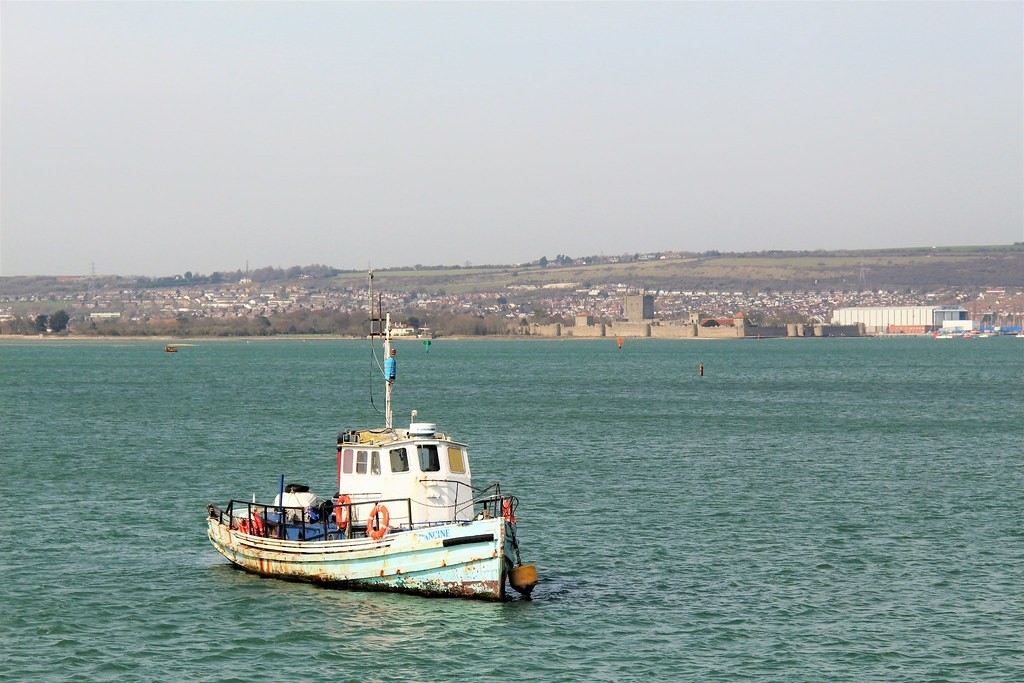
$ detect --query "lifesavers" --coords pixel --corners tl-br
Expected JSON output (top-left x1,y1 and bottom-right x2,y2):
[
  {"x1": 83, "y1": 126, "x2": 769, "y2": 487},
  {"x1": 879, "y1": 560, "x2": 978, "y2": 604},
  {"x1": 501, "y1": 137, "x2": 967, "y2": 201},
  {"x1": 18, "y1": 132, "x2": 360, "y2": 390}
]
[
  {"x1": 336, "y1": 495, "x2": 350, "y2": 529},
  {"x1": 238, "y1": 512, "x2": 265, "y2": 537},
  {"x1": 367, "y1": 505, "x2": 389, "y2": 541},
  {"x1": 502, "y1": 501, "x2": 517, "y2": 525}
]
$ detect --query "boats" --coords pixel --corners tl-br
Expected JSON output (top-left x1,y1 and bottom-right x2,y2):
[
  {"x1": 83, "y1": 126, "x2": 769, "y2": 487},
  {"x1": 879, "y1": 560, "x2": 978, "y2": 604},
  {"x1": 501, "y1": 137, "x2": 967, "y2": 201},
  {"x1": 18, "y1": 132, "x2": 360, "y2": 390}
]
[
  {"x1": 162, "y1": 345, "x2": 179, "y2": 352},
  {"x1": 1015, "y1": 333, "x2": 1024, "y2": 337},
  {"x1": 963, "y1": 333, "x2": 970, "y2": 338},
  {"x1": 979, "y1": 334, "x2": 989, "y2": 338},
  {"x1": 203, "y1": 262, "x2": 540, "y2": 600},
  {"x1": 936, "y1": 331, "x2": 953, "y2": 338}
]
